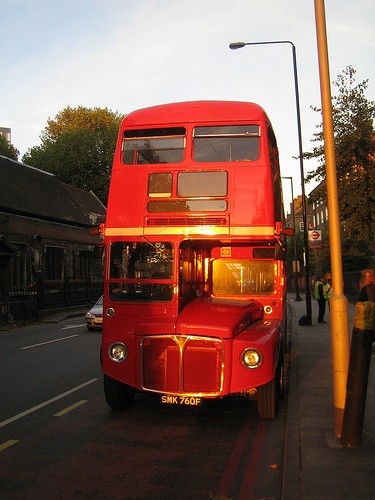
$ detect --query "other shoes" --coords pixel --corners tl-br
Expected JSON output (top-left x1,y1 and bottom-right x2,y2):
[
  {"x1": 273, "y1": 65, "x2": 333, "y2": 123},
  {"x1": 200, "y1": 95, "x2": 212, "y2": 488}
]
[{"x1": 318, "y1": 320, "x2": 328, "y2": 323}]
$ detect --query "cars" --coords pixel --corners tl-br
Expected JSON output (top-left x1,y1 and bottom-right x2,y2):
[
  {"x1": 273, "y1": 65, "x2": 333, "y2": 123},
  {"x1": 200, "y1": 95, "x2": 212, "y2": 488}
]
[{"x1": 86, "y1": 291, "x2": 141, "y2": 330}]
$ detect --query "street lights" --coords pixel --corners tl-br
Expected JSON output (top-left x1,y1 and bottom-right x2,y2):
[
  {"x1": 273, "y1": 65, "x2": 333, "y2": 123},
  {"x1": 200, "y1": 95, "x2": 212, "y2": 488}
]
[{"x1": 228, "y1": 41, "x2": 312, "y2": 328}]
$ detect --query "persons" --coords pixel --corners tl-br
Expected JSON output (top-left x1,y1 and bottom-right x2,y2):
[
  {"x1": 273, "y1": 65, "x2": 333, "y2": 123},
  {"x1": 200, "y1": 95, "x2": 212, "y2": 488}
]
[
  {"x1": 324, "y1": 278, "x2": 332, "y2": 314},
  {"x1": 315, "y1": 276, "x2": 328, "y2": 324}
]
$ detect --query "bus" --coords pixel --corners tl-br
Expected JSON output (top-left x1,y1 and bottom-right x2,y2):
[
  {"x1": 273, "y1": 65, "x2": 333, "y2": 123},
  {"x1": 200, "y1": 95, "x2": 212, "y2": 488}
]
[{"x1": 100, "y1": 98, "x2": 294, "y2": 418}]
